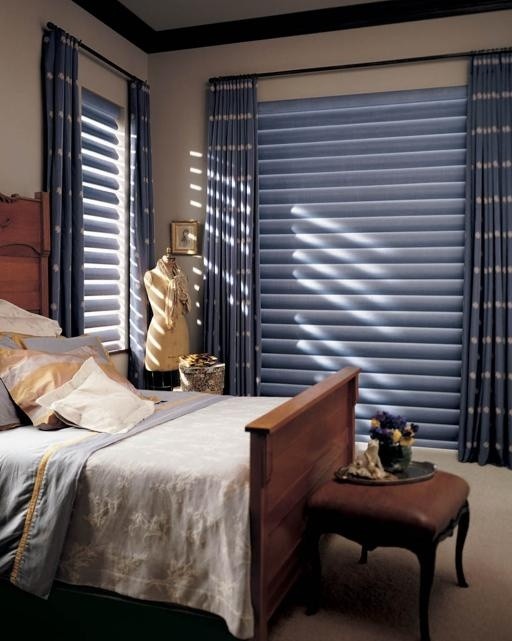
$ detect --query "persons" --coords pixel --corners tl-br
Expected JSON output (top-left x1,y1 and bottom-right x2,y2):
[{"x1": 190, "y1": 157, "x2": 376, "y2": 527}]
[
  {"x1": 143, "y1": 255, "x2": 191, "y2": 372},
  {"x1": 181, "y1": 229, "x2": 192, "y2": 247}
]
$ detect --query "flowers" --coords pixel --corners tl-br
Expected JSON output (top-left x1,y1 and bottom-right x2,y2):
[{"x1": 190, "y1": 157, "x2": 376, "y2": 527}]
[{"x1": 370, "y1": 411, "x2": 419, "y2": 447}]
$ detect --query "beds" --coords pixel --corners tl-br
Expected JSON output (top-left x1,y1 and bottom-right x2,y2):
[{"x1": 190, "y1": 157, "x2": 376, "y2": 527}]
[{"x1": 0, "y1": 191, "x2": 361, "y2": 641}]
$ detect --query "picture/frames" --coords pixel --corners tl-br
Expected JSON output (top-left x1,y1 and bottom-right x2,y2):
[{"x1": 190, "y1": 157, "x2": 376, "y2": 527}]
[{"x1": 168, "y1": 220, "x2": 202, "y2": 256}]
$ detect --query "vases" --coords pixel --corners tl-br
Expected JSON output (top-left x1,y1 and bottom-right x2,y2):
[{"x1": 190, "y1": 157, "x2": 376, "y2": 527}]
[{"x1": 378, "y1": 440, "x2": 412, "y2": 473}]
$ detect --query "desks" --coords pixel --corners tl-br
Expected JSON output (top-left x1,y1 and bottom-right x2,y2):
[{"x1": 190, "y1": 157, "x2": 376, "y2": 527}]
[{"x1": 179, "y1": 362, "x2": 226, "y2": 395}]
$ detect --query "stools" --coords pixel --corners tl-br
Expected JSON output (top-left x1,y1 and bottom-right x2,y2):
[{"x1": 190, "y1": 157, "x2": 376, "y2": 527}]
[{"x1": 304, "y1": 469, "x2": 470, "y2": 641}]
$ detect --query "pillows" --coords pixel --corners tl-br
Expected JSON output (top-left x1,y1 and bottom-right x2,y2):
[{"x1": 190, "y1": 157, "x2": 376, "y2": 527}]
[
  {"x1": 0, "y1": 336, "x2": 30, "y2": 426},
  {"x1": 21, "y1": 336, "x2": 109, "y2": 362},
  {"x1": 36, "y1": 356, "x2": 155, "y2": 433},
  {"x1": 0, "y1": 345, "x2": 160, "y2": 431}
]
